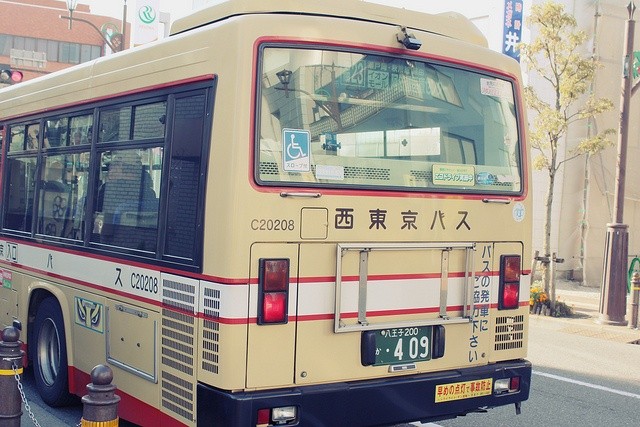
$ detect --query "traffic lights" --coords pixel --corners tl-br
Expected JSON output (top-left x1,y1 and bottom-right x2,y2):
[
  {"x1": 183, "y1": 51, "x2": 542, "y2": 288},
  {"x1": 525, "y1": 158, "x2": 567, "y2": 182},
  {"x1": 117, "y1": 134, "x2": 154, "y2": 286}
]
[{"x1": 0, "y1": 65, "x2": 26, "y2": 84}]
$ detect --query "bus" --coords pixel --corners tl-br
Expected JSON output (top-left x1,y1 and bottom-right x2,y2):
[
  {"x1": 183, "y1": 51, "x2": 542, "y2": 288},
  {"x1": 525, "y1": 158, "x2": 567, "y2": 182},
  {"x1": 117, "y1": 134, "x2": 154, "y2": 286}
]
[{"x1": 0, "y1": 0, "x2": 534, "y2": 426}]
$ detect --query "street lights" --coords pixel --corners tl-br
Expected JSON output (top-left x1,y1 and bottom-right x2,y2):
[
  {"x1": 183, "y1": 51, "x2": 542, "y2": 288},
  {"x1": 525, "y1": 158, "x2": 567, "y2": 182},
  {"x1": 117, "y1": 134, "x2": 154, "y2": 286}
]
[{"x1": 61, "y1": 0, "x2": 127, "y2": 52}]
